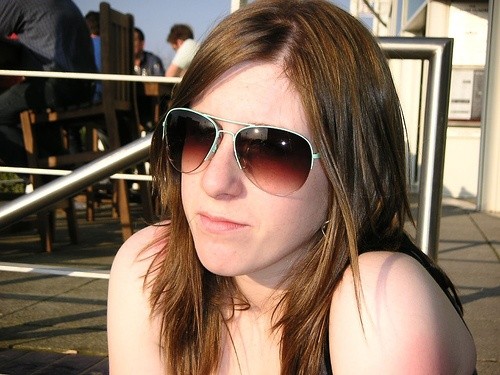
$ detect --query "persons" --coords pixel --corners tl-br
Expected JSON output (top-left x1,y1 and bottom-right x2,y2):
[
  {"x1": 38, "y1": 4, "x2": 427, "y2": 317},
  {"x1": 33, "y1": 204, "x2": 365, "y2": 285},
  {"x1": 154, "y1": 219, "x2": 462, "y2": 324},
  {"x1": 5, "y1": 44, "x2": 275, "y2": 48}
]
[
  {"x1": 105, "y1": 0, "x2": 481, "y2": 375},
  {"x1": 0, "y1": 0, "x2": 201, "y2": 194}
]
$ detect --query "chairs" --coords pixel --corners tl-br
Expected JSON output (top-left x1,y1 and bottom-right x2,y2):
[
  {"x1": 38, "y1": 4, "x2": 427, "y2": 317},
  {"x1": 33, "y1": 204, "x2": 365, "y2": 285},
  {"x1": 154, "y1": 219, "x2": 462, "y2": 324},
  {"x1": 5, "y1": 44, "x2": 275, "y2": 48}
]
[{"x1": 20, "y1": 2, "x2": 155, "y2": 253}]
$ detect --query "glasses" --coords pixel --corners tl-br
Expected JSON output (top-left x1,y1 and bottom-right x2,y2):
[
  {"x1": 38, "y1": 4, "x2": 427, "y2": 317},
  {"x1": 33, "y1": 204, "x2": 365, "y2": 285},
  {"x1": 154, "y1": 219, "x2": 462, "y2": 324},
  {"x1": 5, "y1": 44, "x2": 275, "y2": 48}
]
[{"x1": 162, "y1": 108, "x2": 321, "y2": 195}]
